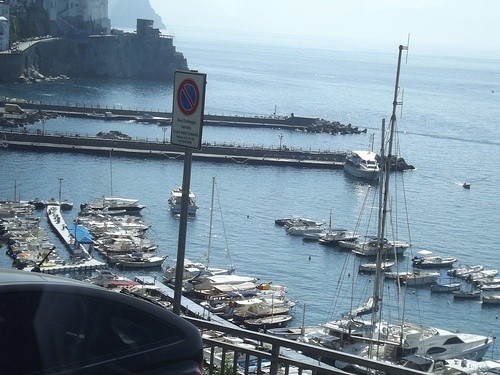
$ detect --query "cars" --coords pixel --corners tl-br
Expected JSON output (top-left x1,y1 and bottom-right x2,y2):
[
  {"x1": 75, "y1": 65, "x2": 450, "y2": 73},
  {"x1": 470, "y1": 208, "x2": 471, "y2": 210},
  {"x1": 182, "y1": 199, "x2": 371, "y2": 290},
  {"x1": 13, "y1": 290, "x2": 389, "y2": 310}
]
[{"x1": 143, "y1": 114, "x2": 153, "y2": 118}]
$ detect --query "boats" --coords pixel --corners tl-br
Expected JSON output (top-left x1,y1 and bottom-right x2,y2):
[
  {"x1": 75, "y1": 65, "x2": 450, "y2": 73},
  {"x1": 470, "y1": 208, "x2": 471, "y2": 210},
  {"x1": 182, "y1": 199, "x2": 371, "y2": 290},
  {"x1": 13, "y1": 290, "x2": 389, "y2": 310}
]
[
  {"x1": 343, "y1": 149, "x2": 381, "y2": 181},
  {"x1": 464, "y1": 182, "x2": 470, "y2": 190},
  {"x1": 0, "y1": 191, "x2": 500, "y2": 375},
  {"x1": 17, "y1": 71, "x2": 71, "y2": 88}
]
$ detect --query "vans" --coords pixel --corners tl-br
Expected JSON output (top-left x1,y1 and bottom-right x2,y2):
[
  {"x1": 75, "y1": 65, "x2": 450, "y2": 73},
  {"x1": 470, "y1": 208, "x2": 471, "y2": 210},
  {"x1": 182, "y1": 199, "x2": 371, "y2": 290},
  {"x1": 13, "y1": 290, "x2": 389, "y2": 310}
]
[{"x1": 5, "y1": 104, "x2": 25, "y2": 114}]
